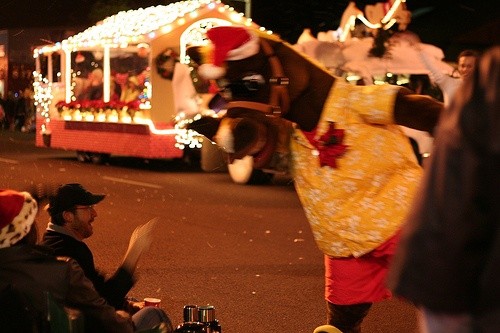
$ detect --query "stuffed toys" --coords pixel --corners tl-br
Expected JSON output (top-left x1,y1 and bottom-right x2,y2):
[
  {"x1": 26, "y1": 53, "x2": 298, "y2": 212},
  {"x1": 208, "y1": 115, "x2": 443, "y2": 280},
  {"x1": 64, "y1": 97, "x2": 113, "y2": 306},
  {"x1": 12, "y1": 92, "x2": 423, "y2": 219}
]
[{"x1": 180, "y1": 27, "x2": 446, "y2": 333}]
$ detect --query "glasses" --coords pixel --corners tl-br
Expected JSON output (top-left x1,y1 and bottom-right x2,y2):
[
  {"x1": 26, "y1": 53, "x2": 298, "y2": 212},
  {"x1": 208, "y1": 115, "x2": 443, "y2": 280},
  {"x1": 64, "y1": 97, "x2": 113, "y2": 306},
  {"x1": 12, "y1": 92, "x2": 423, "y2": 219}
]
[{"x1": 74, "y1": 204, "x2": 96, "y2": 213}]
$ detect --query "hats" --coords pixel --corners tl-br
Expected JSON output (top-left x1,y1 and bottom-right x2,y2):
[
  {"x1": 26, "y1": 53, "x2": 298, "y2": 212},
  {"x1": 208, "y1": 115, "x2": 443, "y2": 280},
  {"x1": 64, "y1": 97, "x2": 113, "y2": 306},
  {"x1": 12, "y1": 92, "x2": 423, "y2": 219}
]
[
  {"x1": 0, "y1": 188, "x2": 38, "y2": 248},
  {"x1": 49, "y1": 183, "x2": 105, "y2": 208}
]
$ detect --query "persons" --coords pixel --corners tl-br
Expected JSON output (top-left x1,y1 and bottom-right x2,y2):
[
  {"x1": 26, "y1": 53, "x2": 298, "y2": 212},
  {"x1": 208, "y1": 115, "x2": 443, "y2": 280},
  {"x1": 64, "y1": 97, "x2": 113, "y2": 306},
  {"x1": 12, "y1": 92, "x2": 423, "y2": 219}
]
[
  {"x1": 384, "y1": 48, "x2": 500, "y2": 333},
  {"x1": 0, "y1": 68, "x2": 141, "y2": 134},
  {"x1": 0, "y1": 184, "x2": 172, "y2": 333}
]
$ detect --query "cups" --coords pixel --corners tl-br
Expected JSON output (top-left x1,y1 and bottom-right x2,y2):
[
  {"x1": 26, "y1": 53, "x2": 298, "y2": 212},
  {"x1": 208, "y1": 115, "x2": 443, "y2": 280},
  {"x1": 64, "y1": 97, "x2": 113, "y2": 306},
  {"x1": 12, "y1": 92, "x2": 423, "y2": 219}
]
[{"x1": 145, "y1": 298, "x2": 161, "y2": 309}]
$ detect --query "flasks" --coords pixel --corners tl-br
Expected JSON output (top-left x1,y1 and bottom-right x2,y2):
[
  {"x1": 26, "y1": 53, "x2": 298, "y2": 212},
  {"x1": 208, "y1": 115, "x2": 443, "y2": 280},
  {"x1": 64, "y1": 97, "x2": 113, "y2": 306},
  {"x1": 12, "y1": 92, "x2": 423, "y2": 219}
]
[
  {"x1": 175, "y1": 304, "x2": 202, "y2": 333},
  {"x1": 199, "y1": 306, "x2": 221, "y2": 333}
]
